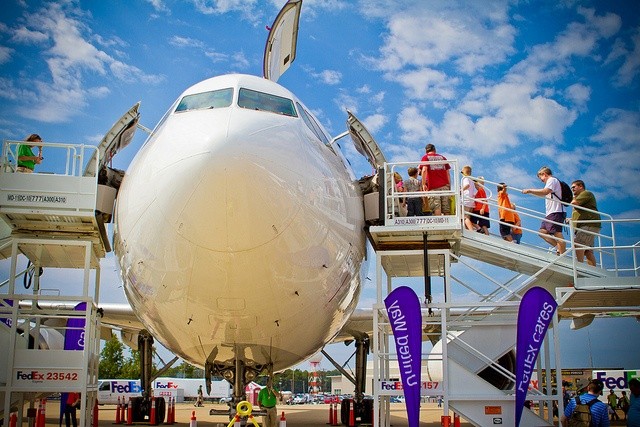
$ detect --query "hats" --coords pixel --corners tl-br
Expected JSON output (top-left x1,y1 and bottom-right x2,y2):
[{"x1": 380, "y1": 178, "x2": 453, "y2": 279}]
[{"x1": 426, "y1": 144, "x2": 435, "y2": 152}]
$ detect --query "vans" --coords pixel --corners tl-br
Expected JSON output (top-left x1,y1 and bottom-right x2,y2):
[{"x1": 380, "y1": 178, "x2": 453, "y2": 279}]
[{"x1": 76, "y1": 380, "x2": 142, "y2": 410}]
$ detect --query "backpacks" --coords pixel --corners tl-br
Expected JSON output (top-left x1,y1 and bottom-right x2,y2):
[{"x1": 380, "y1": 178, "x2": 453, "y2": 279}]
[
  {"x1": 566, "y1": 395, "x2": 602, "y2": 426},
  {"x1": 550, "y1": 176, "x2": 573, "y2": 208}
]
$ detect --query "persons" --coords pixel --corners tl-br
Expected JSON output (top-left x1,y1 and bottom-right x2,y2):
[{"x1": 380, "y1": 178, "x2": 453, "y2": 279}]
[
  {"x1": 576, "y1": 379, "x2": 590, "y2": 395},
  {"x1": 607, "y1": 390, "x2": 620, "y2": 421},
  {"x1": 402, "y1": 167, "x2": 425, "y2": 216},
  {"x1": 15, "y1": 133, "x2": 43, "y2": 173},
  {"x1": 394, "y1": 172, "x2": 408, "y2": 216},
  {"x1": 497, "y1": 182, "x2": 515, "y2": 242},
  {"x1": 510, "y1": 202, "x2": 522, "y2": 244},
  {"x1": 60, "y1": 392, "x2": 68, "y2": 427},
  {"x1": 460, "y1": 166, "x2": 475, "y2": 232},
  {"x1": 619, "y1": 391, "x2": 630, "y2": 420},
  {"x1": 560, "y1": 379, "x2": 610, "y2": 427},
  {"x1": 194, "y1": 385, "x2": 203, "y2": 407},
  {"x1": 65, "y1": 392, "x2": 79, "y2": 427},
  {"x1": 523, "y1": 166, "x2": 573, "y2": 254},
  {"x1": 470, "y1": 176, "x2": 490, "y2": 235},
  {"x1": 555, "y1": 386, "x2": 572, "y2": 412},
  {"x1": 257, "y1": 377, "x2": 280, "y2": 427},
  {"x1": 627, "y1": 378, "x2": 640, "y2": 427},
  {"x1": 565, "y1": 180, "x2": 601, "y2": 266},
  {"x1": 418, "y1": 144, "x2": 451, "y2": 214}
]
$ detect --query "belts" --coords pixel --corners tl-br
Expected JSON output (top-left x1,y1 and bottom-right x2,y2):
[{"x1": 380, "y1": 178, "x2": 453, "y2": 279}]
[{"x1": 264, "y1": 405, "x2": 275, "y2": 408}]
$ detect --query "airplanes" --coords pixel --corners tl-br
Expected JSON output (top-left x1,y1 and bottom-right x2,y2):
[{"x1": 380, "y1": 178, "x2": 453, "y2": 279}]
[{"x1": 0, "y1": 0, "x2": 639, "y2": 427}]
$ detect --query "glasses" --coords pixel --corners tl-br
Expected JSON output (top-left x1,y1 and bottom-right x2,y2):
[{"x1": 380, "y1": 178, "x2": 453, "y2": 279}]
[{"x1": 571, "y1": 186, "x2": 576, "y2": 189}]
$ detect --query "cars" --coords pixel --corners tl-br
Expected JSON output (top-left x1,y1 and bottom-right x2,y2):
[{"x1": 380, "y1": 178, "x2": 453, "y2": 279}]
[{"x1": 285, "y1": 392, "x2": 372, "y2": 405}]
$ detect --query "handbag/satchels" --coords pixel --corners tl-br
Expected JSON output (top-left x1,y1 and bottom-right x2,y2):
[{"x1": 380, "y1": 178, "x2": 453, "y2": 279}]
[{"x1": 76, "y1": 402, "x2": 81, "y2": 410}]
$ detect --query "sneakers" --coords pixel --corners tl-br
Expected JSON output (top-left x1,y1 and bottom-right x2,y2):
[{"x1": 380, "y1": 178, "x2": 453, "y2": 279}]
[{"x1": 476, "y1": 228, "x2": 485, "y2": 234}]
